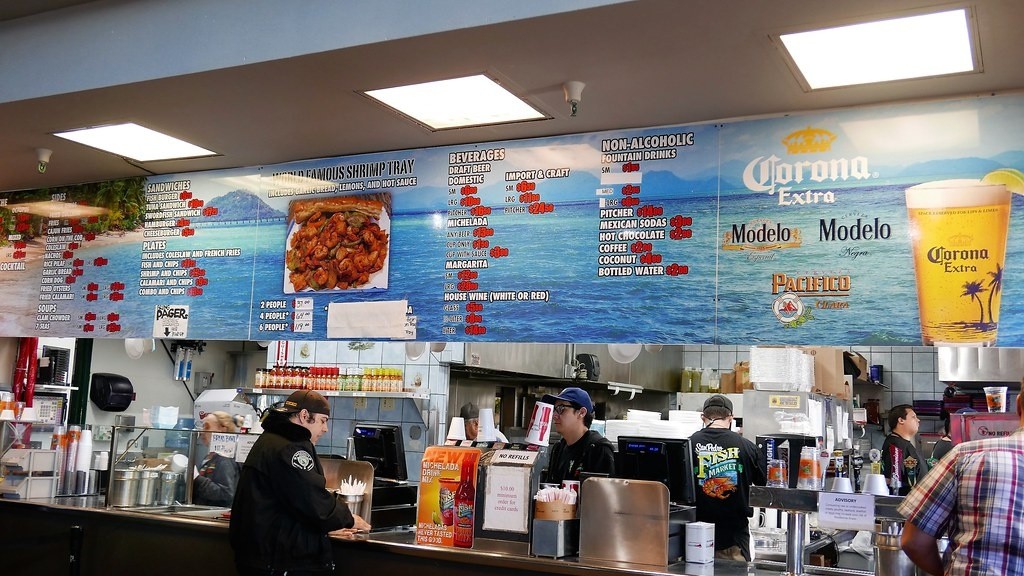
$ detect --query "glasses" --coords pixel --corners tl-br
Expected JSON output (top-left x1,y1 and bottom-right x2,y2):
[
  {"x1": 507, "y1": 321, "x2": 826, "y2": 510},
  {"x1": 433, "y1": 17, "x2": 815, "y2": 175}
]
[{"x1": 553, "y1": 404, "x2": 582, "y2": 414}]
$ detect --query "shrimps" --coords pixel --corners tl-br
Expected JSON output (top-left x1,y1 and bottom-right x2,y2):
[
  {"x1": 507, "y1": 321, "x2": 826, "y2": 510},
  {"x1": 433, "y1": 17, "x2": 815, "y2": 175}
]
[{"x1": 285, "y1": 212, "x2": 389, "y2": 293}]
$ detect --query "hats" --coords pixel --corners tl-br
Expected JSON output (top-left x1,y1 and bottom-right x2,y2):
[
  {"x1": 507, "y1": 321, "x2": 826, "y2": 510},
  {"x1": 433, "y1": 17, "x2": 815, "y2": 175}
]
[
  {"x1": 542, "y1": 387, "x2": 593, "y2": 415},
  {"x1": 703, "y1": 395, "x2": 733, "y2": 413},
  {"x1": 956, "y1": 407, "x2": 978, "y2": 414},
  {"x1": 459, "y1": 401, "x2": 479, "y2": 420},
  {"x1": 273, "y1": 389, "x2": 330, "y2": 416}
]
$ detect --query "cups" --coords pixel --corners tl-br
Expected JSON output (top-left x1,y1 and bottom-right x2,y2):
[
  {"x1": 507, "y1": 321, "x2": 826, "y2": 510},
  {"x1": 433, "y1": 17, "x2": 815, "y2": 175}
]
[
  {"x1": 905, "y1": 185, "x2": 1012, "y2": 347},
  {"x1": 438, "y1": 477, "x2": 459, "y2": 526},
  {"x1": 447, "y1": 416, "x2": 466, "y2": 441},
  {"x1": 476, "y1": 409, "x2": 495, "y2": 442},
  {"x1": 766, "y1": 460, "x2": 787, "y2": 489},
  {"x1": 525, "y1": 401, "x2": 554, "y2": 446},
  {"x1": 984, "y1": 387, "x2": 1007, "y2": 412},
  {"x1": 797, "y1": 447, "x2": 821, "y2": 490},
  {"x1": 50, "y1": 425, "x2": 108, "y2": 495}
]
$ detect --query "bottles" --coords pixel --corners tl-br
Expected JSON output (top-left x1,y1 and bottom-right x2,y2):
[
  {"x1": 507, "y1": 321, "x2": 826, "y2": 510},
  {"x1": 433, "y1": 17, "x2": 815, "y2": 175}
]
[
  {"x1": 682, "y1": 367, "x2": 720, "y2": 393},
  {"x1": 452, "y1": 460, "x2": 474, "y2": 547},
  {"x1": 254, "y1": 367, "x2": 403, "y2": 393}
]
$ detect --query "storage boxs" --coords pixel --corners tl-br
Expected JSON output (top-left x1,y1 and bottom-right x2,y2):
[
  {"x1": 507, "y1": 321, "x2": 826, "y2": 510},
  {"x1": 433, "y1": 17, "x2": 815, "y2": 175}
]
[
  {"x1": 720, "y1": 346, "x2": 884, "y2": 424},
  {"x1": 193, "y1": 388, "x2": 253, "y2": 429},
  {"x1": 685, "y1": 521, "x2": 715, "y2": 564}
]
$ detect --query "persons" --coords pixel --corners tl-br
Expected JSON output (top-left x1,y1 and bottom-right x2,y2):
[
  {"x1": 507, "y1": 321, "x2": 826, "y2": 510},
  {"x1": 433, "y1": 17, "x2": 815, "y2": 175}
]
[
  {"x1": 897, "y1": 376, "x2": 1024, "y2": 576},
  {"x1": 881, "y1": 404, "x2": 929, "y2": 496},
  {"x1": 929, "y1": 407, "x2": 978, "y2": 471},
  {"x1": 184, "y1": 411, "x2": 245, "y2": 507},
  {"x1": 230, "y1": 391, "x2": 372, "y2": 576},
  {"x1": 690, "y1": 395, "x2": 767, "y2": 562},
  {"x1": 543, "y1": 388, "x2": 616, "y2": 498},
  {"x1": 462, "y1": 403, "x2": 478, "y2": 440}
]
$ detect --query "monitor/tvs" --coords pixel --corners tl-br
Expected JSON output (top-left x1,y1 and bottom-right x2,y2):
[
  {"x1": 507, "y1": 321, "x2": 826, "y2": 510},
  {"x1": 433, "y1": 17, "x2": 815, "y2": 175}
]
[
  {"x1": 354, "y1": 422, "x2": 409, "y2": 481},
  {"x1": 618, "y1": 435, "x2": 696, "y2": 505}
]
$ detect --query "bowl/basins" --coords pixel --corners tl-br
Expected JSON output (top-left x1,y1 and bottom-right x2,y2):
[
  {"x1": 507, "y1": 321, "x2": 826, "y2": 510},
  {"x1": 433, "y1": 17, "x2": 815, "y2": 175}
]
[
  {"x1": 172, "y1": 454, "x2": 188, "y2": 472},
  {"x1": 831, "y1": 478, "x2": 853, "y2": 494},
  {"x1": 0, "y1": 391, "x2": 37, "y2": 421},
  {"x1": 862, "y1": 474, "x2": 888, "y2": 496}
]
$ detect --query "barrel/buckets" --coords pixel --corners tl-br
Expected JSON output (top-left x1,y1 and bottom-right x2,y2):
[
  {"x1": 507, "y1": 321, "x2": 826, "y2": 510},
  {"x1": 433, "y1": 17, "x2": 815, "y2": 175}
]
[
  {"x1": 872, "y1": 533, "x2": 916, "y2": 576},
  {"x1": 138, "y1": 470, "x2": 159, "y2": 504},
  {"x1": 113, "y1": 469, "x2": 141, "y2": 506},
  {"x1": 159, "y1": 473, "x2": 185, "y2": 506},
  {"x1": 336, "y1": 490, "x2": 364, "y2": 515}
]
